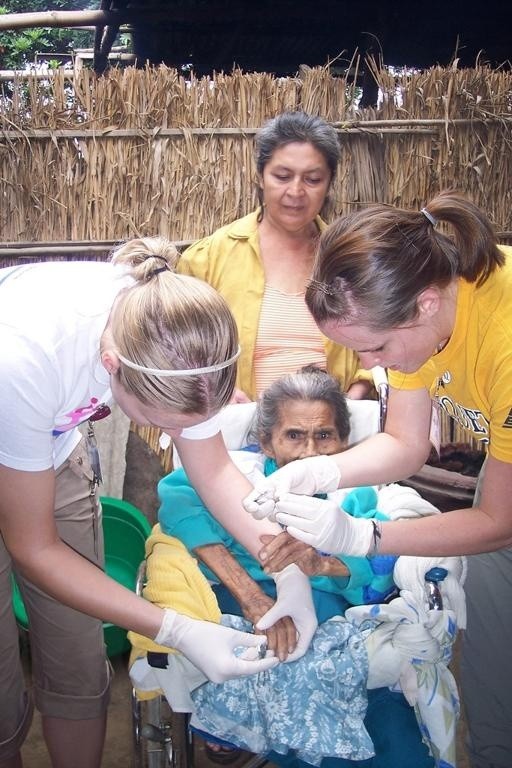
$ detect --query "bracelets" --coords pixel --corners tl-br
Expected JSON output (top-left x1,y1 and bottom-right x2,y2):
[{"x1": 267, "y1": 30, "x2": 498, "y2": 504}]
[{"x1": 368, "y1": 518, "x2": 383, "y2": 559}]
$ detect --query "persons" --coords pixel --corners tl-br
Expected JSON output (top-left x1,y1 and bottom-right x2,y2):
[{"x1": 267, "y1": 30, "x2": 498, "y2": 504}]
[
  {"x1": 175, "y1": 111, "x2": 375, "y2": 762},
  {"x1": 2, "y1": 238, "x2": 318, "y2": 768},
  {"x1": 242, "y1": 190, "x2": 512, "y2": 768}
]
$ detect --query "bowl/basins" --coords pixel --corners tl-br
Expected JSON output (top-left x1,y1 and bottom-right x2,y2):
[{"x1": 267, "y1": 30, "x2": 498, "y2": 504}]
[{"x1": 14, "y1": 496, "x2": 151, "y2": 661}]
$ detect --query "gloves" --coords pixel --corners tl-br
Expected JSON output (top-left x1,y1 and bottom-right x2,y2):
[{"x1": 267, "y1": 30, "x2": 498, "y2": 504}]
[
  {"x1": 243, "y1": 453, "x2": 374, "y2": 558},
  {"x1": 153, "y1": 562, "x2": 318, "y2": 684}
]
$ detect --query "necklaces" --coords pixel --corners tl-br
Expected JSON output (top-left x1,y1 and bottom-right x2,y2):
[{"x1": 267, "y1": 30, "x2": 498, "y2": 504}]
[{"x1": 438, "y1": 343, "x2": 443, "y2": 352}]
